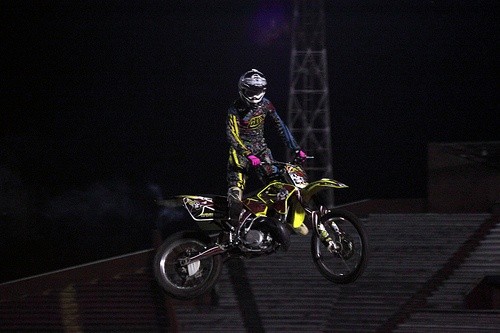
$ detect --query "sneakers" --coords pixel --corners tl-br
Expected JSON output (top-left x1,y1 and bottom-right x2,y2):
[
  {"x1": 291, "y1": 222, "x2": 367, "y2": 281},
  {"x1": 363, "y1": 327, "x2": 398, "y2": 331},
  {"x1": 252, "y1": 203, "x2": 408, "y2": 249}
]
[{"x1": 220, "y1": 219, "x2": 240, "y2": 247}]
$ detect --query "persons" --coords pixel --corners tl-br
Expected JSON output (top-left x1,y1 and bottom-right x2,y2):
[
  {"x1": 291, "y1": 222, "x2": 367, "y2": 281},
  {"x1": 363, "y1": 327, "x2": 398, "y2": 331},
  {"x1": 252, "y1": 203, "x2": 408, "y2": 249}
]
[{"x1": 224, "y1": 67, "x2": 310, "y2": 242}]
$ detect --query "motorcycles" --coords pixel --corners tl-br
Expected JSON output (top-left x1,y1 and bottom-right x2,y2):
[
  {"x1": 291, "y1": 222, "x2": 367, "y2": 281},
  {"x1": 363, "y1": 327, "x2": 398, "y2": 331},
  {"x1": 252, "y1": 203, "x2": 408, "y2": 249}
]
[{"x1": 150, "y1": 148, "x2": 369, "y2": 302}]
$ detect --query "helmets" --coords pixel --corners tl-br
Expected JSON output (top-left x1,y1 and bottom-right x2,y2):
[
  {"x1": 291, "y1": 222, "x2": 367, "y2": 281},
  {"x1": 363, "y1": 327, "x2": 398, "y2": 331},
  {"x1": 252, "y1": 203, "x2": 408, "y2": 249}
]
[{"x1": 238, "y1": 69, "x2": 267, "y2": 103}]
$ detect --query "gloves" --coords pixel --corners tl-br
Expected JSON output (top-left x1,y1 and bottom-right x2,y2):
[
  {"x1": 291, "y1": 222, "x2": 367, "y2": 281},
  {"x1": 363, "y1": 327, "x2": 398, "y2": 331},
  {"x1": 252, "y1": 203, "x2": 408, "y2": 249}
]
[
  {"x1": 246, "y1": 154, "x2": 260, "y2": 171},
  {"x1": 290, "y1": 150, "x2": 306, "y2": 164}
]
